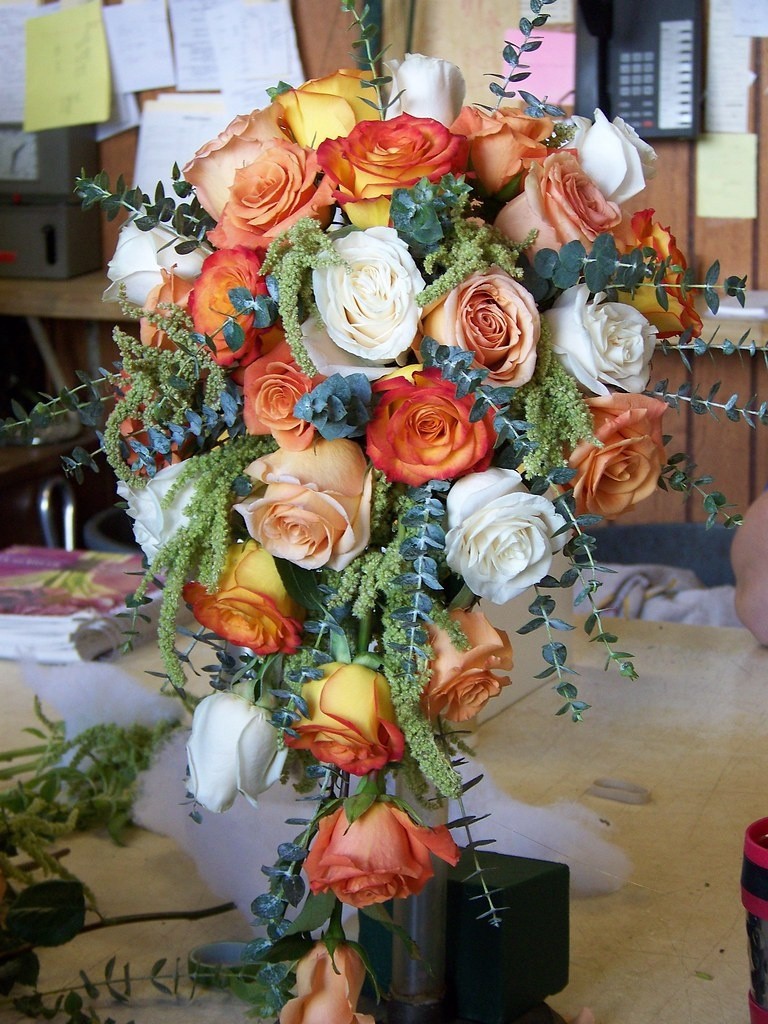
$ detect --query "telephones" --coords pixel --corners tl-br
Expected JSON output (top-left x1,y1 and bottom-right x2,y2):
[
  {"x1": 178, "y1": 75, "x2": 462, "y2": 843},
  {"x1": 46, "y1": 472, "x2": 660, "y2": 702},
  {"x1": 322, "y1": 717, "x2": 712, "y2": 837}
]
[{"x1": 574, "y1": 0, "x2": 704, "y2": 140}]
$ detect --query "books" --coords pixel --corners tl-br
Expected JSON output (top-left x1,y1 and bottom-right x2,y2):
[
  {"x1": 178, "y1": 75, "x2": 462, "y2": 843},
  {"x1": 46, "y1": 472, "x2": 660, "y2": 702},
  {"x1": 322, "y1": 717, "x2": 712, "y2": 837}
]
[
  {"x1": 719, "y1": 291, "x2": 768, "y2": 314},
  {"x1": 0, "y1": 545, "x2": 195, "y2": 664}
]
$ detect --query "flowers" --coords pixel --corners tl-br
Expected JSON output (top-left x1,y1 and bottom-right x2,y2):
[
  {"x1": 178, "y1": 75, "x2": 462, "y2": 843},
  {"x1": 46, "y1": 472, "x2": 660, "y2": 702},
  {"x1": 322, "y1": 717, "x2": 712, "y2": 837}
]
[{"x1": 1, "y1": 0, "x2": 768, "y2": 1015}]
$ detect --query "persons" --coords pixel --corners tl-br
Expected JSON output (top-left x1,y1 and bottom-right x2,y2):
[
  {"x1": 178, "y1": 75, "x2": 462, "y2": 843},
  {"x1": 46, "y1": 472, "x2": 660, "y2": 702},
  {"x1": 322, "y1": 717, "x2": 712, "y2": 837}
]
[{"x1": 730, "y1": 483, "x2": 768, "y2": 647}]
